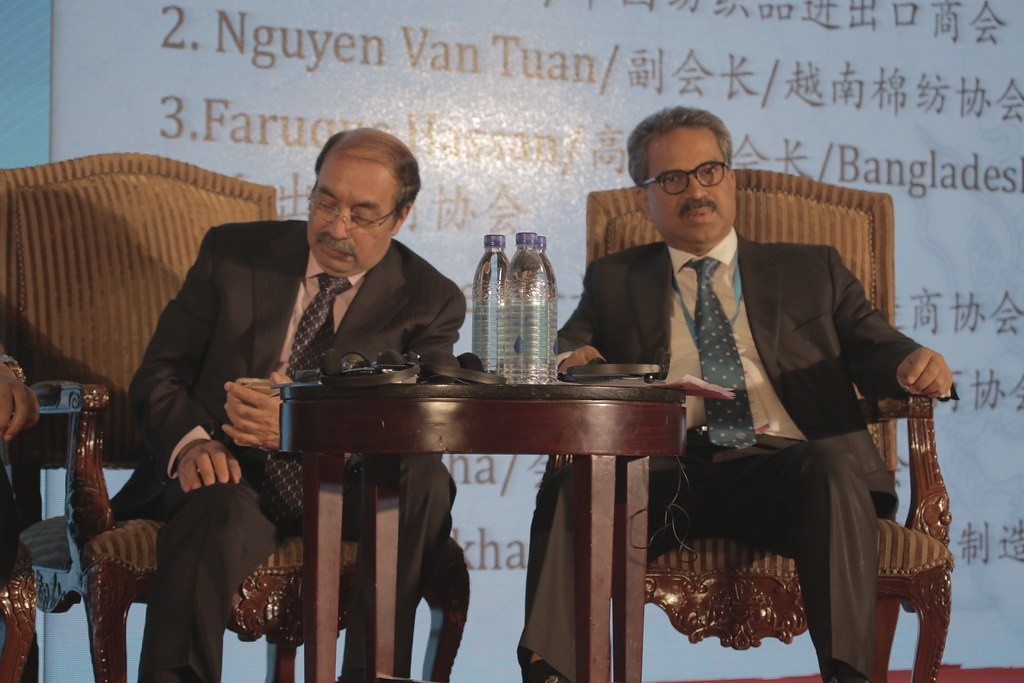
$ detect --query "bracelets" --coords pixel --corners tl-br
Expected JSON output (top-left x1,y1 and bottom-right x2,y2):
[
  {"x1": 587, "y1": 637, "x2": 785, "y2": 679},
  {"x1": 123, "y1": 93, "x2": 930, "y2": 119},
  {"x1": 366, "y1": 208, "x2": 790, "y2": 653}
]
[{"x1": 1, "y1": 353, "x2": 27, "y2": 382}]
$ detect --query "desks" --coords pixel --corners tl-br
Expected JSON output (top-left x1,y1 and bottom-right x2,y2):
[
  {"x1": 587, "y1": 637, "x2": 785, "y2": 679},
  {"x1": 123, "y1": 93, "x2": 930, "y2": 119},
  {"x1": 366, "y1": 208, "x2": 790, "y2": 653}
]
[{"x1": 278, "y1": 385, "x2": 689, "y2": 683}]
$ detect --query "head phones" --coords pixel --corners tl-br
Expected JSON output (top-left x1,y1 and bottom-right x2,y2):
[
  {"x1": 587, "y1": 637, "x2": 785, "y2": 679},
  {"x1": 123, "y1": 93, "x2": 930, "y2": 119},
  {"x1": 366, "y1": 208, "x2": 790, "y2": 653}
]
[
  {"x1": 567, "y1": 349, "x2": 671, "y2": 381},
  {"x1": 319, "y1": 348, "x2": 507, "y2": 389}
]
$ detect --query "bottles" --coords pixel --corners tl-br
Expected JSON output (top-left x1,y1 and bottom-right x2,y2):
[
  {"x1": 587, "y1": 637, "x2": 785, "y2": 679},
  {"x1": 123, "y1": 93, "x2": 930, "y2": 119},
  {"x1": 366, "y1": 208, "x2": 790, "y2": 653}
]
[
  {"x1": 535, "y1": 237, "x2": 558, "y2": 383},
  {"x1": 504, "y1": 232, "x2": 549, "y2": 385},
  {"x1": 472, "y1": 234, "x2": 510, "y2": 377}
]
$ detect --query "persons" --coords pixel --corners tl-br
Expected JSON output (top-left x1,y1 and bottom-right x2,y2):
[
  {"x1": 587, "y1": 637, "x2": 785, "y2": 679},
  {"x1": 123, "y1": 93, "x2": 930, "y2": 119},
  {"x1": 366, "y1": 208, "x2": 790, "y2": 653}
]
[
  {"x1": 1, "y1": 343, "x2": 41, "y2": 681},
  {"x1": 517, "y1": 107, "x2": 954, "y2": 683},
  {"x1": 110, "y1": 128, "x2": 467, "y2": 683}
]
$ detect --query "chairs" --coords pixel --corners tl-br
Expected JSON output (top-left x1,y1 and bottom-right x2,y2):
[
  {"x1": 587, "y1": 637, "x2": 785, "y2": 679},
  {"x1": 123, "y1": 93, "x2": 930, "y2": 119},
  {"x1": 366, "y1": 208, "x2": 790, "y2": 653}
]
[
  {"x1": 547, "y1": 170, "x2": 952, "y2": 683},
  {"x1": 0, "y1": 153, "x2": 471, "y2": 683}
]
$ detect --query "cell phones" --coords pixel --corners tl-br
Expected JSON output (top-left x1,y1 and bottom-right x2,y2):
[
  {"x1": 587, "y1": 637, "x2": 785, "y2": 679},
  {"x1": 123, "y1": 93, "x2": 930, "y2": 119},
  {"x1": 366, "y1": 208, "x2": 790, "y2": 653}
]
[{"x1": 233, "y1": 377, "x2": 276, "y2": 446}]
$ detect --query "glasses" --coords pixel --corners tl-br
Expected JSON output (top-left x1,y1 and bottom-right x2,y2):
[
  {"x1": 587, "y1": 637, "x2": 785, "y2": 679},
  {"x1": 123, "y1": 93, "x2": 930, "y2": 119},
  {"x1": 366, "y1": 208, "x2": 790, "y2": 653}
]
[
  {"x1": 641, "y1": 161, "x2": 731, "y2": 196},
  {"x1": 306, "y1": 185, "x2": 399, "y2": 230}
]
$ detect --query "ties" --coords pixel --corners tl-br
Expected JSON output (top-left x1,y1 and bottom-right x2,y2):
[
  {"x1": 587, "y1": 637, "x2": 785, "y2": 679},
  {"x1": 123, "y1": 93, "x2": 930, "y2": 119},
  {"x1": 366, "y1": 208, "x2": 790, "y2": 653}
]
[
  {"x1": 682, "y1": 255, "x2": 759, "y2": 450},
  {"x1": 263, "y1": 275, "x2": 353, "y2": 523}
]
